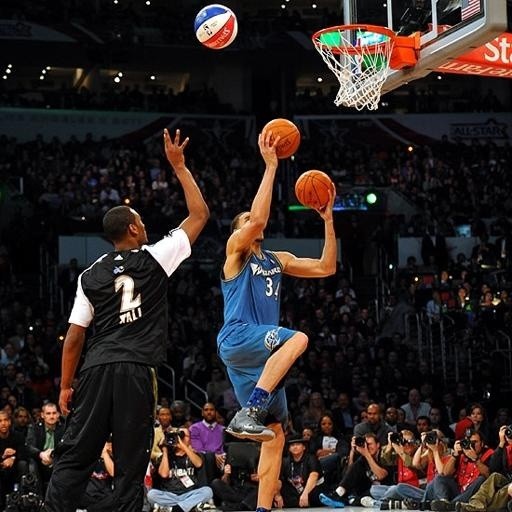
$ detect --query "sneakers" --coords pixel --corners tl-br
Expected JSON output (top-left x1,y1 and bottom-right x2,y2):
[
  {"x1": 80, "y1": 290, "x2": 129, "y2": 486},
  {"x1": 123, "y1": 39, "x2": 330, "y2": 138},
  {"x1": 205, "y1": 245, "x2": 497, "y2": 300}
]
[
  {"x1": 319, "y1": 491, "x2": 345, "y2": 508},
  {"x1": 152, "y1": 501, "x2": 205, "y2": 511},
  {"x1": 360, "y1": 496, "x2": 486, "y2": 511},
  {"x1": 225, "y1": 408, "x2": 276, "y2": 443}
]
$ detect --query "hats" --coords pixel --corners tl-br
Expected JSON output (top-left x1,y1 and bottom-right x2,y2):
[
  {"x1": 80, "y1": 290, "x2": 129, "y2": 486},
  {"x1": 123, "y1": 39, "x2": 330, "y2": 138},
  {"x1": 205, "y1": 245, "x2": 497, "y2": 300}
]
[{"x1": 288, "y1": 434, "x2": 309, "y2": 444}]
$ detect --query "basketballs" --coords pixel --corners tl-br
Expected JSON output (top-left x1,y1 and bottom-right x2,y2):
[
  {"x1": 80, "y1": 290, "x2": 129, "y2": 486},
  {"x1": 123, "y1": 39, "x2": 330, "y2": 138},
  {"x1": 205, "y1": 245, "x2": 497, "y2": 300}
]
[
  {"x1": 263, "y1": 118, "x2": 301, "y2": 159},
  {"x1": 295, "y1": 170, "x2": 334, "y2": 209},
  {"x1": 194, "y1": 4, "x2": 238, "y2": 49}
]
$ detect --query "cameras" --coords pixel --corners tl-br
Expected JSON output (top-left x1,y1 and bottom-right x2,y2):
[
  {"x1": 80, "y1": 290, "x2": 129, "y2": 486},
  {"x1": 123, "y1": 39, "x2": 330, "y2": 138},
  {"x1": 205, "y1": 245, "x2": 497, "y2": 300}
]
[
  {"x1": 390, "y1": 432, "x2": 406, "y2": 447},
  {"x1": 460, "y1": 426, "x2": 475, "y2": 451},
  {"x1": 425, "y1": 430, "x2": 438, "y2": 445},
  {"x1": 355, "y1": 435, "x2": 366, "y2": 448},
  {"x1": 505, "y1": 424, "x2": 512, "y2": 439}
]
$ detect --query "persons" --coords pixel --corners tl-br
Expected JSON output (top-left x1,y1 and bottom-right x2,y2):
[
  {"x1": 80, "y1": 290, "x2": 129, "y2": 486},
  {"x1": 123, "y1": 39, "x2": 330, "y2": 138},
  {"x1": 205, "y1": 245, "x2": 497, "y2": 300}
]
[{"x1": 1, "y1": 0, "x2": 512, "y2": 512}]
